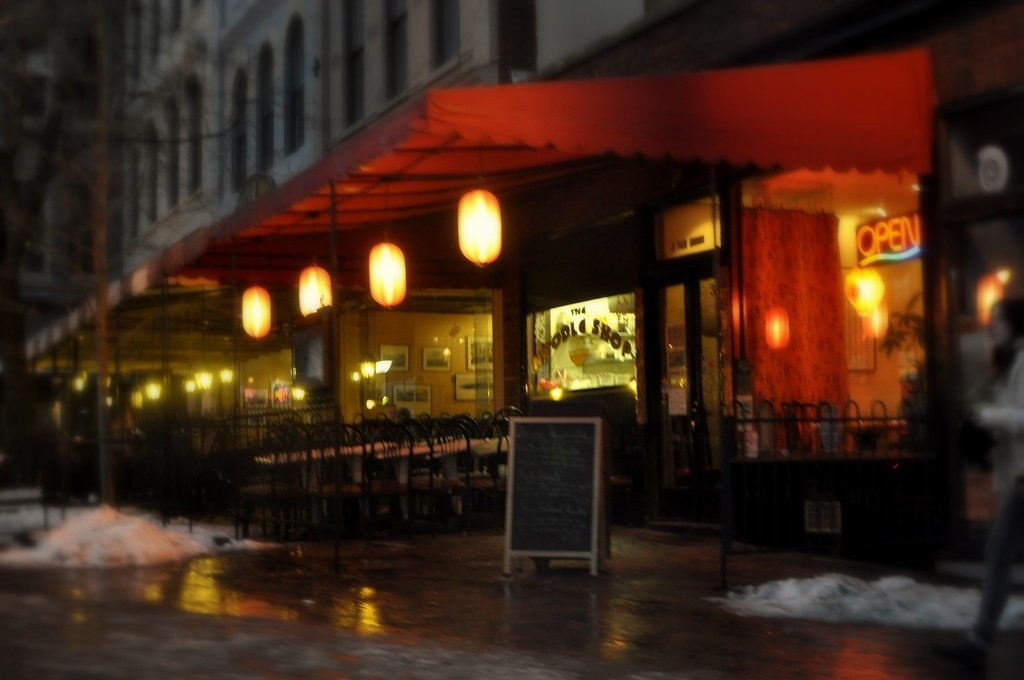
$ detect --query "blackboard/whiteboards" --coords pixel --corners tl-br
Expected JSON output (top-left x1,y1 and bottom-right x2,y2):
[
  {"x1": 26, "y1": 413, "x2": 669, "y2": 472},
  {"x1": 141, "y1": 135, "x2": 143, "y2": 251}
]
[{"x1": 504, "y1": 415, "x2": 603, "y2": 558}]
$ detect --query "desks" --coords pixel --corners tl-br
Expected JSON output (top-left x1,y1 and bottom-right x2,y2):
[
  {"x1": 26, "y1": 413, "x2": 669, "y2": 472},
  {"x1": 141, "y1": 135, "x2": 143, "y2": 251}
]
[
  {"x1": 255, "y1": 435, "x2": 510, "y2": 470},
  {"x1": 842, "y1": 419, "x2": 908, "y2": 452}
]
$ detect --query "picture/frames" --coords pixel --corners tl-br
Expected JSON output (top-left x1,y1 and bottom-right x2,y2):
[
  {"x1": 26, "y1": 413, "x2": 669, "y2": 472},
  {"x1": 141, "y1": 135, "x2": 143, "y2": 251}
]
[{"x1": 378, "y1": 336, "x2": 494, "y2": 417}]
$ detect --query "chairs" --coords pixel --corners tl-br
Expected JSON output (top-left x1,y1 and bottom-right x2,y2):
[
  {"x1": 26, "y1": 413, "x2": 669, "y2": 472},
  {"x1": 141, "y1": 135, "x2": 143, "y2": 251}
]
[
  {"x1": 159, "y1": 403, "x2": 502, "y2": 545},
  {"x1": 490, "y1": 406, "x2": 525, "y2": 437},
  {"x1": 780, "y1": 403, "x2": 823, "y2": 453}
]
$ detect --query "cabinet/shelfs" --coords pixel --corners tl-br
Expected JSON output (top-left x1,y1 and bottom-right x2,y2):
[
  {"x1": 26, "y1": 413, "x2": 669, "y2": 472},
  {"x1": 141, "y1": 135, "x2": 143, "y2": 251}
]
[{"x1": 583, "y1": 332, "x2": 636, "y2": 369}]
